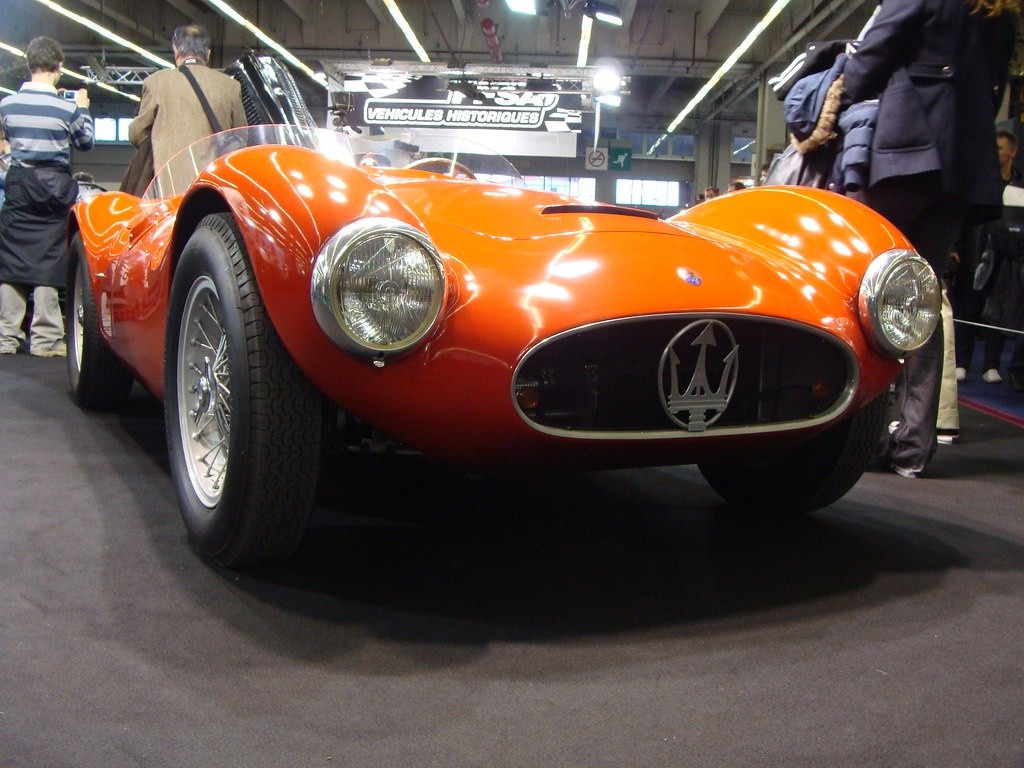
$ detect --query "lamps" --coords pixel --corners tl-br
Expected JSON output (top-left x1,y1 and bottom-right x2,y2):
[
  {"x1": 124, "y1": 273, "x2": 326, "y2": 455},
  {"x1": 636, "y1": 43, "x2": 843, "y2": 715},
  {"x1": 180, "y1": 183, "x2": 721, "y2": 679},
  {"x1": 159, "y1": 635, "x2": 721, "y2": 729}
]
[{"x1": 589, "y1": 2, "x2": 623, "y2": 26}]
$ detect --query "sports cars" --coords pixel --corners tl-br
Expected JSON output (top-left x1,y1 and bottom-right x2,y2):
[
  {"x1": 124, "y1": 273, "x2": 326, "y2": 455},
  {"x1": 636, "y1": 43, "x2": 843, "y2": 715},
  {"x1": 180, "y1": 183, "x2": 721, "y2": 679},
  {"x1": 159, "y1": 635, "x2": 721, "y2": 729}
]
[{"x1": 65, "y1": 124, "x2": 942, "y2": 562}]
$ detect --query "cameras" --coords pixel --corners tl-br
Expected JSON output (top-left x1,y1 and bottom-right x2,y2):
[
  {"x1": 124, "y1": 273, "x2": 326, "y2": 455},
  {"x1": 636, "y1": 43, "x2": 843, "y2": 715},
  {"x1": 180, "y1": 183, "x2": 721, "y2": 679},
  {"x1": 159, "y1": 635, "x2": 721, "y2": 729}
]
[{"x1": 63, "y1": 90, "x2": 79, "y2": 101}]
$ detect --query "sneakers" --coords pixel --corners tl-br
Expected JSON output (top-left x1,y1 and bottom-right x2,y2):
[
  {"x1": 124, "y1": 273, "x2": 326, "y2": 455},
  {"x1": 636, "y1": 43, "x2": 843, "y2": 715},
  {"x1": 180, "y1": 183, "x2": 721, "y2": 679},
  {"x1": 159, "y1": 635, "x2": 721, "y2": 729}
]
[
  {"x1": 29, "y1": 341, "x2": 68, "y2": 358},
  {"x1": 0, "y1": 343, "x2": 16, "y2": 354}
]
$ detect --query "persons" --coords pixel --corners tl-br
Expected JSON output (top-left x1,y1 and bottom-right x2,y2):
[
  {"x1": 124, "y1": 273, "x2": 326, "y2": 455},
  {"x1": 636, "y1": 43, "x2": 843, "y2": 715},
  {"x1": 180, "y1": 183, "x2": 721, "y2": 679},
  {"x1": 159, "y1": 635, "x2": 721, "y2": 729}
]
[
  {"x1": 842, "y1": 0, "x2": 1024, "y2": 479},
  {"x1": 119, "y1": 25, "x2": 248, "y2": 199},
  {"x1": 0, "y1": 36, "x2": 95, "y2": 358},
  {"x1": 692, "y1": 164, "x2": 771, "y2": 204}
]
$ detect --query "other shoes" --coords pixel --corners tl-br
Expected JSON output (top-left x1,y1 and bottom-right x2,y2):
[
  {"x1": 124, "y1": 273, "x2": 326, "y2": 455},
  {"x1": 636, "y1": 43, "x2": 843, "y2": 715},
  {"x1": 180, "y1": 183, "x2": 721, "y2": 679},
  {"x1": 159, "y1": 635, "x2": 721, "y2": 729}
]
[{"x1": 890, "y1": 463, "x2": 916, "y2": 479}]
